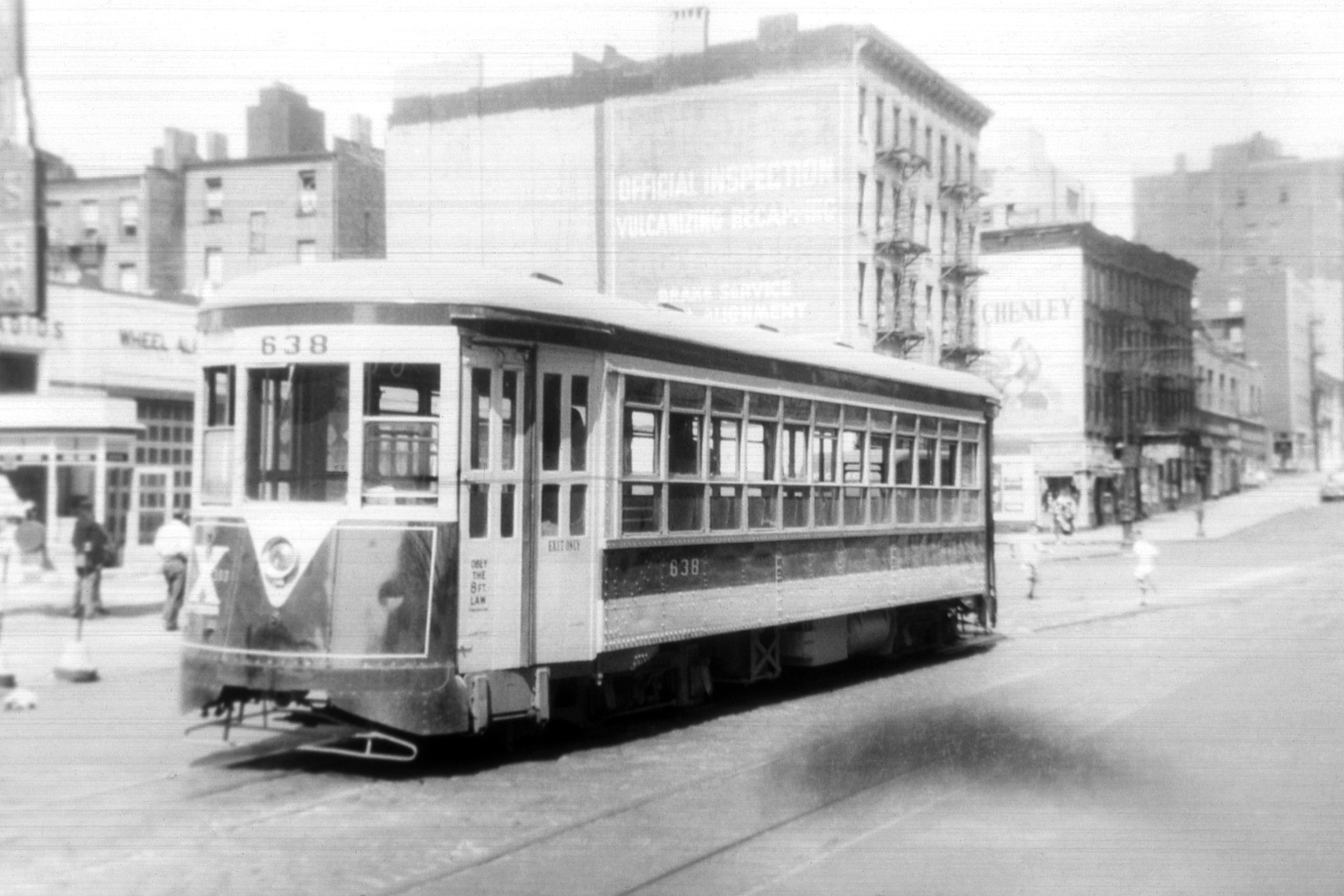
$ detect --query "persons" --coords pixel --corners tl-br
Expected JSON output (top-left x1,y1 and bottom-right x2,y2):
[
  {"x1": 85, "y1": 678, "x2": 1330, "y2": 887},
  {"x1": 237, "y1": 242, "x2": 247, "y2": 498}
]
[
  {"x1": 1, "y1": 497, "x2": 50, "y2": 599},
  {"x1": 153, "y1": 506, "x2": 195, "y2": 632},
  {"x1": 518, "y1": 378, "x2": 718, "y2": 541},
  {"x1": 70, "y1": 500, "x2": 111, "y2": 617}
]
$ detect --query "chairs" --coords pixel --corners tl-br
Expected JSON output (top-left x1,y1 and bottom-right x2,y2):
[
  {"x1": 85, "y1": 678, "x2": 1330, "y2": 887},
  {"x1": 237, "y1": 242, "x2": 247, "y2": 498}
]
[{"x1": 667, "y1": 493, "x2": 979, "y2": 532}]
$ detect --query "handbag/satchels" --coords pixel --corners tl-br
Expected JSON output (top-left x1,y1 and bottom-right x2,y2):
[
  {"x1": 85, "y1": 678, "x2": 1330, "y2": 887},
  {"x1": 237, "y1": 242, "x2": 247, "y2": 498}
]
[{"x1": 100, "y1": 541, "x2": 118, "y2": 567}]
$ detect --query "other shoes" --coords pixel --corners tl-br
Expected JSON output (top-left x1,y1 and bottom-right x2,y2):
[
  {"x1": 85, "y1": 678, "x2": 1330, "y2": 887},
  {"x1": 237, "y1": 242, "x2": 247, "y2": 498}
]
[
  {"x1": 70, "y1": 605, "x2": 85, "y2": 617},
  {"x1": 166, "y1": 624, "x2": 179, "y2": 630},
  {"x1": 92, "y1": 605, "x2": 111, "y2": 617}
]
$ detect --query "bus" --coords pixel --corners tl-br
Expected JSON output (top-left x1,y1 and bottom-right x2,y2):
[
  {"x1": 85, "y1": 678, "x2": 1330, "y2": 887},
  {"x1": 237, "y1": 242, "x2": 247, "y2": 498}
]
[{"x1": 178, "y1": 266, "x2": 1003, "y2": 762}]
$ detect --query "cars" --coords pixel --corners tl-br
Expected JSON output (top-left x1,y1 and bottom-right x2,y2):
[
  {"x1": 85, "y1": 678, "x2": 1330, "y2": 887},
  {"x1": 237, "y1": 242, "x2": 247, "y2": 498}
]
[{"x1": 1322, "y1": 482, "x2": 1343, "y2": 499}]
